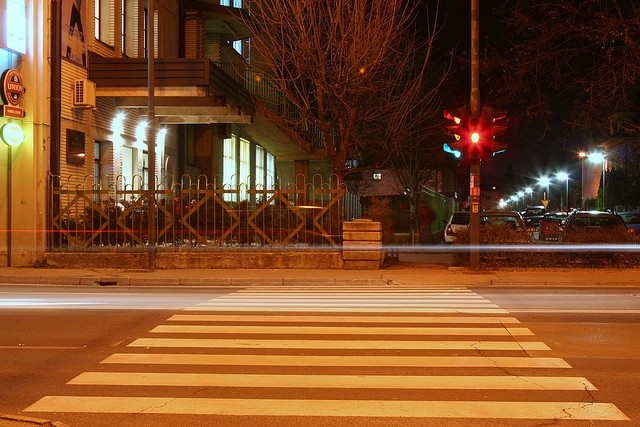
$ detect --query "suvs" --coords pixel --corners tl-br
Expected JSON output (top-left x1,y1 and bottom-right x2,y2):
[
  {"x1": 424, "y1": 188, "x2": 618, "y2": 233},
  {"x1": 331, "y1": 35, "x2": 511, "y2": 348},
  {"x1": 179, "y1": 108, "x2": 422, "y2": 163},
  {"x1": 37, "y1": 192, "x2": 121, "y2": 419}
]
[
  {"x1": 559, "y1": 208, "x2": 633, "y2": 244},
  {"x1": 522, "y1": 206, "x2": 548, "y2": 227}
]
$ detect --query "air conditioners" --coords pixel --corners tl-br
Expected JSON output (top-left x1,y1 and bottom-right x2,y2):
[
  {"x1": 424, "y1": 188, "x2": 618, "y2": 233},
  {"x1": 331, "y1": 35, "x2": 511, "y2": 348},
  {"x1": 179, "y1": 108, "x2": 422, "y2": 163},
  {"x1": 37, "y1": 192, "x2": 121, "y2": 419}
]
[{"x1": 73, "y1": 79, "x2": 97, "y2": 107}]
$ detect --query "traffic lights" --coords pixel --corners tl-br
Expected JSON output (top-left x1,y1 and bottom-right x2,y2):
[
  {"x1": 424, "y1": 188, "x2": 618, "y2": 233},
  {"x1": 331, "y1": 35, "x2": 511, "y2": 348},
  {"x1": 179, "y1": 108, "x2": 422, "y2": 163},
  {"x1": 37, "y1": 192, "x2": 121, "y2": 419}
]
[
  {"x1": 442, "y1": 104, "x2": 471, "y2": 161},
  {"x1": 468, "y1": 116, "x2": 483, "y2": 160},
  {"x1": 480, "y1": 106, "x2": 508, "y2": 156}
]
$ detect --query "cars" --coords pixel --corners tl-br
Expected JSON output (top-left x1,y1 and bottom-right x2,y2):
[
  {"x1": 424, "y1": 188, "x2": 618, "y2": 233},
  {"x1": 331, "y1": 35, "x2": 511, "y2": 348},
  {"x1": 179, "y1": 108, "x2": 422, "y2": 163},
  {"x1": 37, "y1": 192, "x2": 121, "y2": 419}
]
[
  {"x1": 555, "y1": 210, "x2": 573, "y2": 225},
  {"x1": 614, "y1": 209, "x2": 640, "y2": 234},
  {"x1": 537, "y1": 213, "x2": 565, "y2": 241},
  {"x1": 466, "y1": 210, "x2": 535, "y2": 261},
  {"x1": 443, "y1": 210, "x2": 471, "y2": 243}
]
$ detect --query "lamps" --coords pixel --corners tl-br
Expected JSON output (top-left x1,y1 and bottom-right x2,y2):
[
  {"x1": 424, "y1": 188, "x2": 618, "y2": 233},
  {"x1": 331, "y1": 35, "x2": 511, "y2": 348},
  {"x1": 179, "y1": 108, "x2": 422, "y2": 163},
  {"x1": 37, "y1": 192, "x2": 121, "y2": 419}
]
[{"x1": 2, "y1": 122, "x2": 24, "y2": 147}]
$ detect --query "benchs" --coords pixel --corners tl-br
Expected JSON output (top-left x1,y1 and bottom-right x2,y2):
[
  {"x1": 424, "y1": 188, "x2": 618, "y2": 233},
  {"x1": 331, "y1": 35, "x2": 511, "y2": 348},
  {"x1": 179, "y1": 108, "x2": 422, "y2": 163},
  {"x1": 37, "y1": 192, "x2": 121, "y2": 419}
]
[{"x1": 394, "y1": 233, "x2": 419, "y2": 246}]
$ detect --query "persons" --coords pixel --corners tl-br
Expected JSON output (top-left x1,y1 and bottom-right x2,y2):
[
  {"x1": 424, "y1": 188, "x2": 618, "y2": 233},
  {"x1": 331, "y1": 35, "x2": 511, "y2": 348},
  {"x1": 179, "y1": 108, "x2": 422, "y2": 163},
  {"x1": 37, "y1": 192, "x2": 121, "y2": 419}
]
[{"x1": 410, "y1": 200, "x2": 436, "y2": 244}]
